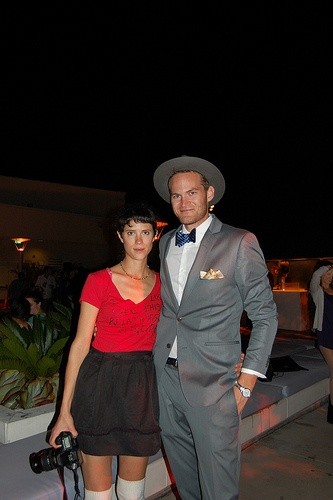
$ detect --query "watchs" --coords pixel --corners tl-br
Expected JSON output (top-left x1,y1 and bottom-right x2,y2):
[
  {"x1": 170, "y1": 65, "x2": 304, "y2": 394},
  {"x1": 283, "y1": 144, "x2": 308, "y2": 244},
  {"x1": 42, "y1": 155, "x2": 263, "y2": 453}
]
[{"x1": 234, "y1": 382, "x2": 255, "y2": 399}]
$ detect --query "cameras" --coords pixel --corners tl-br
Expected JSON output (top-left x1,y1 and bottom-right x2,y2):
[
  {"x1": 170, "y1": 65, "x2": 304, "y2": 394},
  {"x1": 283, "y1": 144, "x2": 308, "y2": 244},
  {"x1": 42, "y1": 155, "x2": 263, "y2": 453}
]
[{"x1": 29, "y1": 431, "x2": 83, "y2": 474}]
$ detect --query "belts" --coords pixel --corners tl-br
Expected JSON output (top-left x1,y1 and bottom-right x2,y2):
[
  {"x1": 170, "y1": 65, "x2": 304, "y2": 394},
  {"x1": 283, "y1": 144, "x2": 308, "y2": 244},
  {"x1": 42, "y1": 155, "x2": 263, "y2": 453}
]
[{"x1": 166, "y1": 357, "x2": 178, "y2": 367}]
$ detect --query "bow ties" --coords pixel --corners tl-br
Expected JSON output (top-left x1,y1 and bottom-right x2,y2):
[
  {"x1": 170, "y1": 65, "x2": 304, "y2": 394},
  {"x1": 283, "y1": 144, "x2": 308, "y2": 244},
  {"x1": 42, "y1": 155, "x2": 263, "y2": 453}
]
[{"x1": 174, "y1": 228, "x2": 196, "y2": 247}]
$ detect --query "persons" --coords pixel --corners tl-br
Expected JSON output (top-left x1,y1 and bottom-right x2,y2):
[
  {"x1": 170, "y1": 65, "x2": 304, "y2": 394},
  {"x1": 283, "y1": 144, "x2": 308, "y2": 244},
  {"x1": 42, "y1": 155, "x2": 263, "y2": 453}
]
[
  {"x1": 154, "y1": 156, "x2": 280, "y2": 500},
  {"x1": 49, "y1": 212, "x2": 163, "y2": 500},
  {"x1": 7, "y1": 265, "x2": 80, "y2": 333},
  {"x1": 308, "y1": 260, "x2": 333, "y2": 424}
]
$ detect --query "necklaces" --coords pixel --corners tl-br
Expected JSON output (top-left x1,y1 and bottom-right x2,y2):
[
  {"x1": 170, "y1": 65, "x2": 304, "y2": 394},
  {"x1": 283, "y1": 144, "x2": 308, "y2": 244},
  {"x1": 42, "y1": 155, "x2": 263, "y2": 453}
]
[{"x1": 120, "y1": 260, "x2": 150, "y2": 280}]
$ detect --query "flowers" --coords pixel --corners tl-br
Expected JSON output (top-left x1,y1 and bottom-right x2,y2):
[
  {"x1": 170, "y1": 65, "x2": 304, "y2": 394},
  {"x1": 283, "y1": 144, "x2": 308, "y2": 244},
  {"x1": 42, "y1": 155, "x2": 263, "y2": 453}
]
[{"x1": 277, "y1": 261, "x2": 289, "y2": 278}]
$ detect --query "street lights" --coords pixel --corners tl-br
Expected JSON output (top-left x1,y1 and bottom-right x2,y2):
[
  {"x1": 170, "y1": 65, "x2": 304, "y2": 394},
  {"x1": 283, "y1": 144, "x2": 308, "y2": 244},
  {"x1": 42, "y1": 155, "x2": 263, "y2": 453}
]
[{"x1": 12, "y1": 236, "x2": 31, "y2": 274}]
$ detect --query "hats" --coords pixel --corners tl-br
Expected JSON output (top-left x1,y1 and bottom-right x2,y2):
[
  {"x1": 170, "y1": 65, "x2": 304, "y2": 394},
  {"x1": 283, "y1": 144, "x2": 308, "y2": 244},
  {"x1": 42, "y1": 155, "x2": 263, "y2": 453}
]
[{"x1": 151, "y1": 155, "x2": 226, "y2": 207}]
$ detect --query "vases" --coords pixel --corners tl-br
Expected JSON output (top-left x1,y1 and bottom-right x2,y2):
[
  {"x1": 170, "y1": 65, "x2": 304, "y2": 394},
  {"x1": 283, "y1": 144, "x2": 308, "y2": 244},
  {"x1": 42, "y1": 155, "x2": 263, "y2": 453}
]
[{"x1": 279, "y1": 278, "x2": 286, "y2": 290}]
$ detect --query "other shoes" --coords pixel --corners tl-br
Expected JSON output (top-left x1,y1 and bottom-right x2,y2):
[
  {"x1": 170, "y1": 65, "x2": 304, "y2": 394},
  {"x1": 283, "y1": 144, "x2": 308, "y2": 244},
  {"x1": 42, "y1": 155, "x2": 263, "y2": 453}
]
[{"x1": 326, "y1": 403, "x2": 332, "y2": 423}]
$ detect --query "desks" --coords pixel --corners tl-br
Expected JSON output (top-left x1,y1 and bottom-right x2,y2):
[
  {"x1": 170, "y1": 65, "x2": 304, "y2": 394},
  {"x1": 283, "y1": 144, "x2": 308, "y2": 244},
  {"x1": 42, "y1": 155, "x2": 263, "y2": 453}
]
[{"x1": 273, "y1": 289, "x2": 311, "y2": 331}]
